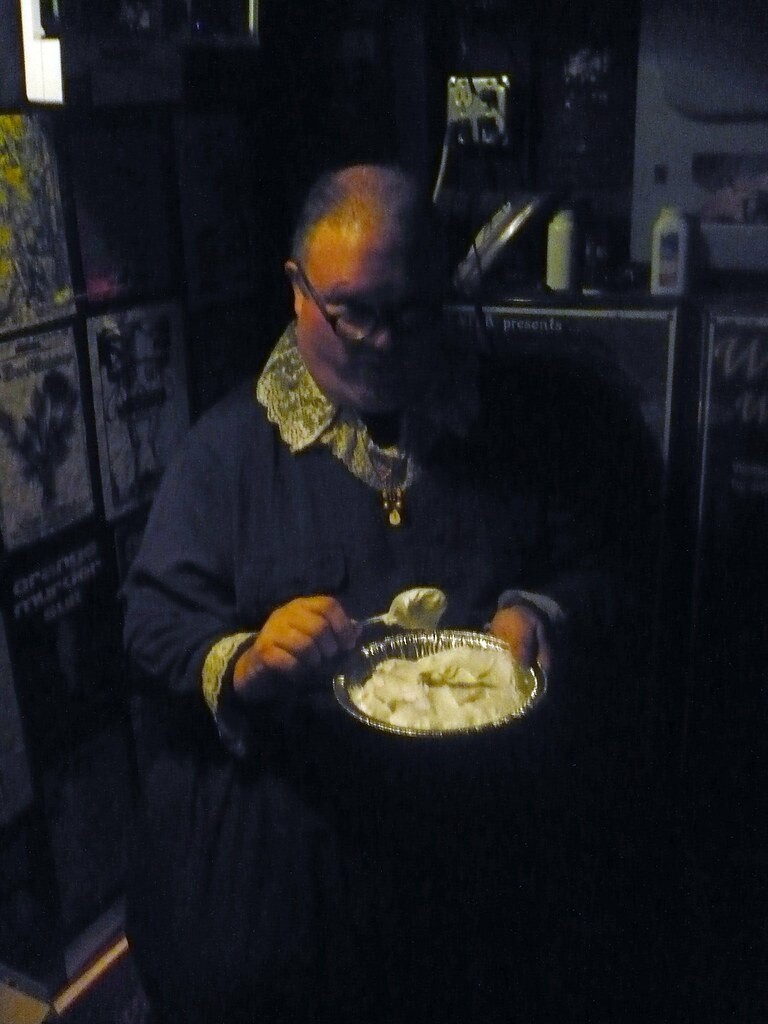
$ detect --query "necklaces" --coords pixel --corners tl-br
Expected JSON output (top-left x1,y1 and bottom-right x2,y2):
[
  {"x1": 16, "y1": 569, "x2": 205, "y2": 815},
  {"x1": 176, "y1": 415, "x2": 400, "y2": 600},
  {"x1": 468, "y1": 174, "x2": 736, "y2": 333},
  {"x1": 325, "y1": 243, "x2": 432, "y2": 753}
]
[{"x1": 358, "y1": 431, "x2": 401, "y2": 527}]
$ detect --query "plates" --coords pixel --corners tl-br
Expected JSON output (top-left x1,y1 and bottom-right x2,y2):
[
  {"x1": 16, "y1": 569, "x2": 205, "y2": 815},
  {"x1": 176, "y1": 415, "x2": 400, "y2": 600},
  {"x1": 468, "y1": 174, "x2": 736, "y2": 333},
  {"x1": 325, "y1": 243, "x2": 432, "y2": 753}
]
[{"x1": 331, "y1": 635, "x2": 548, "y2": 738}]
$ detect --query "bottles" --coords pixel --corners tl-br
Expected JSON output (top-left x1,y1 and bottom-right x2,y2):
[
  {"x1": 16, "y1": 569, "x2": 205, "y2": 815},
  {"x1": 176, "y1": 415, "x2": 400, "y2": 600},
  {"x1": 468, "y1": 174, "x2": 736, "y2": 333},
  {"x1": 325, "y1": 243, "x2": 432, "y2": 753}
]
[
  {"x1": 545, "y1": 207, "x2": 580, "y2": 293},
  {"x1": 648, "y1": 205, "x2": 689, "y2": 298}
]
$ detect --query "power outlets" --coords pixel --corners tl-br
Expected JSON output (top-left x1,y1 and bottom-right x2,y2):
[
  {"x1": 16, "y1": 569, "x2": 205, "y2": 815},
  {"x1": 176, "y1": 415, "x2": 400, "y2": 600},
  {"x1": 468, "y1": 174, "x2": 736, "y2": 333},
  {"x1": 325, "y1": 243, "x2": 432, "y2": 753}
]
[{"x1": 448, "y1": 75, "x2": 511, "y2": 147}]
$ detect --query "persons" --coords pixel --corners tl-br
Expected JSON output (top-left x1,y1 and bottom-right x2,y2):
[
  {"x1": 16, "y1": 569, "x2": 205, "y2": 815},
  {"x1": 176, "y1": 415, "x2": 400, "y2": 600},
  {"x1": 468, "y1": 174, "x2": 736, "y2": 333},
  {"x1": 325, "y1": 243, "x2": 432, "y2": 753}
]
[{"x1": 119, "y1": 164, "x2": 594, "y2": 1024}]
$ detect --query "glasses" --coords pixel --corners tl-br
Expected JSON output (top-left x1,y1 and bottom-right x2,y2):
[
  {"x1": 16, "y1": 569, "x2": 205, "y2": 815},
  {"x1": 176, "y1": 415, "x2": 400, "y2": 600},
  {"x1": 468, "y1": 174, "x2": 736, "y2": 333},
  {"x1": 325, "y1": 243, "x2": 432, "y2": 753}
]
[{"x1": 294, "y1": 258, "x2": 451, "y2": 346}]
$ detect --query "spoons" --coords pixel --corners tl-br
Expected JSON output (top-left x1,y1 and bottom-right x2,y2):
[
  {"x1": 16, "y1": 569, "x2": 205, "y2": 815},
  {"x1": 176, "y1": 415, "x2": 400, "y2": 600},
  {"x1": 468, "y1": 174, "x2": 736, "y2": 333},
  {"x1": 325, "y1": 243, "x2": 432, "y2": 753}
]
[{"x1": 352, "y1": 588, "x2": 447, "y2": 630}]
